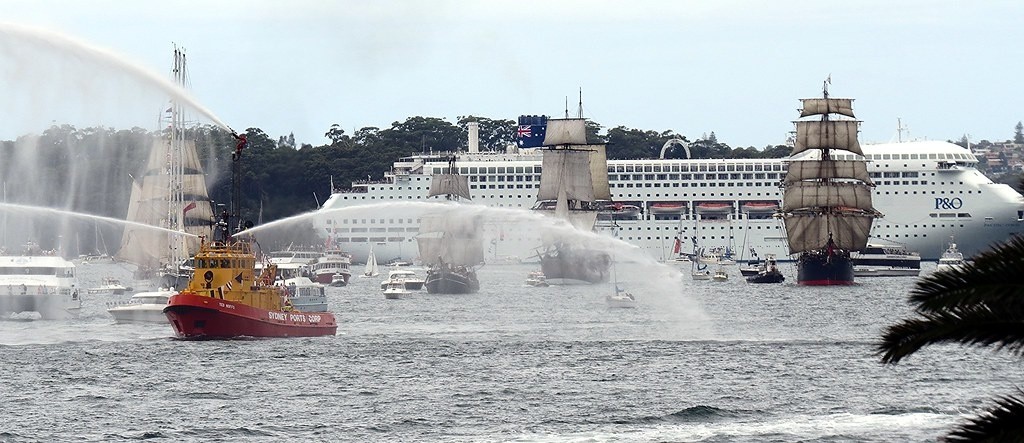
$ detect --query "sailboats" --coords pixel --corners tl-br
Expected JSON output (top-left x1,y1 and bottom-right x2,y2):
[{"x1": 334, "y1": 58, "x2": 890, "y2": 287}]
[
  {"x1": 739, "y1": 210, "x2": 785, "y2": 283},
  {"x1": 691, "y1": 220, "x2": 709, "y2": 279},
  {"x1": 782, "y1": 79, "x2": 881, "y2": 286},
  {"x1": 606, "y1": 254, "x2": 638, "y2": 307},
  {"x1": 526, "y1": 87, "x2": 613, "y2": 284},
  {"x1": 416, "y1": 154, "x2": 486, "y2": 294},
  {"x1": 653, "y1": 212, "x2": 739, "y2": 265},
  {"x1": 111, "y1": 37, "x2": 222, "y2": 292},
  {"x1": 359, "y1": 246, "x2": 379, "y2": 279}
]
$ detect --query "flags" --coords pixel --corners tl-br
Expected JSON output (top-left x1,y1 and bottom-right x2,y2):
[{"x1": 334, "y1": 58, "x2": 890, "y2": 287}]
[
  {"x1": 517, "y1": 125, "x2": 531, "y2": 138},
  {"x1": 226, "y1": 280, "x2": 232, "y2": 290}
]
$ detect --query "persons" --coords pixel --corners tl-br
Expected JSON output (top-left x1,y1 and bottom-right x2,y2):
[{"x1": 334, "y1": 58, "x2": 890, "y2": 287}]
[
  {"x1": 885, "y1": 249, "x2": 907, "y2": 255},
  {"x1": 70, "y1": 285, "x2": 76, "y2": 296},
  {"x1": 767, "y1": 262, "x2": 773, "y2": 273},
  {"x1": 8, "y1": 285, "x2": 12, "y2": 292},
  {"x1": 38, "y1": 285, "x2": 48, "y2": 294},
  {"x1": 19, "y1": 283, "x2": 27, "y2": 294},
  {"x1": 235, "y1": 272, "x2": 242, "y2": 283},
  {"x1": 310, "y1": 270, "x2": 316, "y2": 277},
  {"x1": 811, "y1": 248, "x2": 816, "y2": 252},
  {"x1": 303, "y1": 270, "x2": 308, "y2": 277},
  {"x1": 285, "y1": 299, "x2": 291, "y2": 306},
  {"x1": 66, "y1": 271, "x2": 71, "y2": 278},
  {"x1": 276, "y1": 282, "x2": 296, "y2": 297}
]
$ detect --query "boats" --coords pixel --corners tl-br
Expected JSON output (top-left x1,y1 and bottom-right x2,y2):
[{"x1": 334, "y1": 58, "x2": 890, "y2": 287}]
[
  {"x1": 936, "y1": 238, "x2": 967, "y2": 274},
  {"x1": 380, "y1": 267, "x2": 425, "y2": 289},
  {"x1": 0, "y1": 257, "x2": 81, "y2": 314},
  {"x1": 714, "y1": 267, "x2": 729, "y2": 282},
  {"x1": 88, "y1": 280, "x2": 132, "y2": 295},
  {"x1": 312, "y1": 218, "x2": 351, "y2": 286},
  {"x1": 251, "y1": 244, "x2": 328, "y2": 278},
  {"x1": 105, "y1": 287, "x2": 184, "y2": 322},
  {"x1": 852, "y1": 243, "x2": 921, "y2": 276},
  {"x1": 383, "y1": 279, "x2": 412, "y2": 299},
  {"x1": 0, "y1": 220, "x2": 110, "y2": 264},
  {"x1": 276, "y1": 275, "x2": 328, "y2": 311},
  {"x1": 525, "y1": 270, "x2": 547, "y2": 285},
  {"x1": 162, "y1": 128, "x2": 337, "y2": 336},
  {"x1": 385, "y1": 255, "x2": 413, "y2": 265}
]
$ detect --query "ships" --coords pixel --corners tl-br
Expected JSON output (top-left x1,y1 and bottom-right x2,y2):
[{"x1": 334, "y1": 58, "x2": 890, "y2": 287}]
[{"x1": 318, "y1": 121, "x2": 1024, "y2": 265}]
